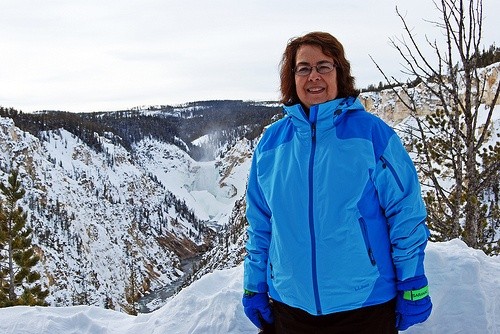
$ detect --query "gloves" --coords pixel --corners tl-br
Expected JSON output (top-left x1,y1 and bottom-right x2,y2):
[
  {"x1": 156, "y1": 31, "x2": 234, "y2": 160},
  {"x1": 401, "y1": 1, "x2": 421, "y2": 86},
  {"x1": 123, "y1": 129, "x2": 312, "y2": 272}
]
[
  {"x1": 394, "y1": 275, "x2": 433, "y2": 331},
  {"x1": 242, "y1": 290, "x2": 274, "y2": 331}
]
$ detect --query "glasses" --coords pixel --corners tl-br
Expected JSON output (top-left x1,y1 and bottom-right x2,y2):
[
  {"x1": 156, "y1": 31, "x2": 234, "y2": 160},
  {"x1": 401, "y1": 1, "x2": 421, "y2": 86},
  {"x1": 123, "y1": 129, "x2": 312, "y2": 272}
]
[{"x1": 291, "y1": 62, "x2": 337, "y2": 76}]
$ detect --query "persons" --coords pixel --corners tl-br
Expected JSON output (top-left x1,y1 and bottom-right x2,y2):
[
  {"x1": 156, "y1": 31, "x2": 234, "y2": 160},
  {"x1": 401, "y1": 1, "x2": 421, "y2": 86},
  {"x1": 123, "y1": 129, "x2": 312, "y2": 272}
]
[{"x1": 243, "y1": 32, "x2": 433, "y2": 334}]
